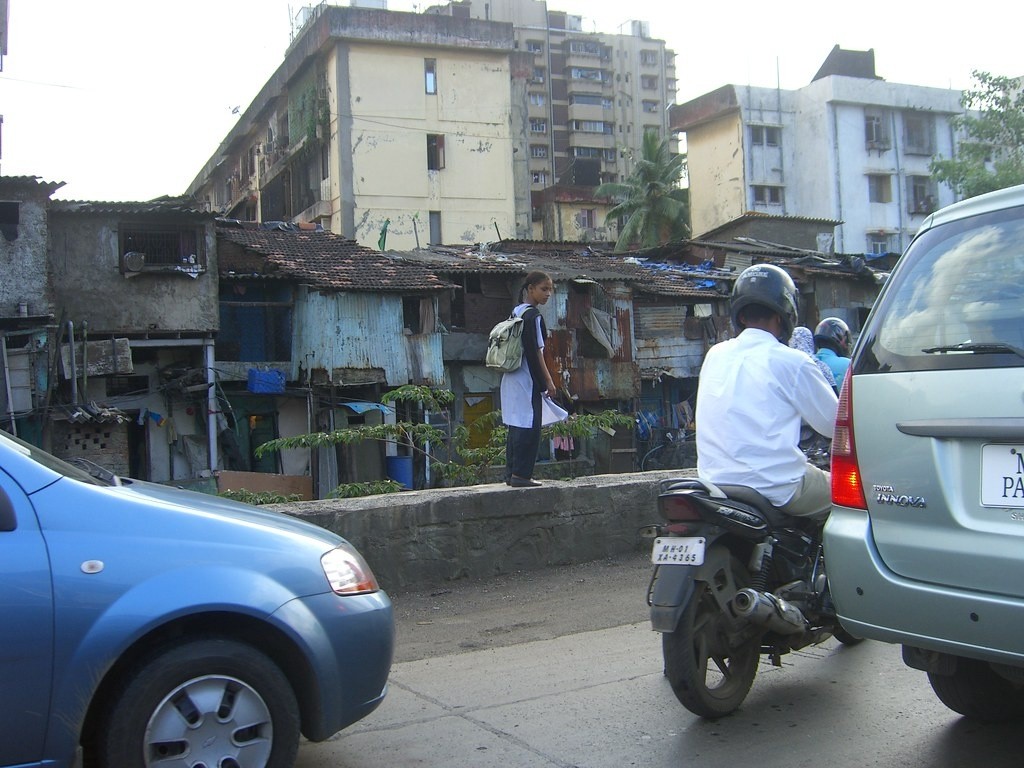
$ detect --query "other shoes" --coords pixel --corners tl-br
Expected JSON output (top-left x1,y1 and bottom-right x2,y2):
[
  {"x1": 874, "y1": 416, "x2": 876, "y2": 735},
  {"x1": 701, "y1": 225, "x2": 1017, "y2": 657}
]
[
  {"x1": 506, "y1": 476, "x2": 511, "y2": 485},
  {"x1": 818, "y1": 575, "x2": 836, "y2": 619},
  {"x1": 510, "y1": 476, "x2": 542, "y2": 487}
]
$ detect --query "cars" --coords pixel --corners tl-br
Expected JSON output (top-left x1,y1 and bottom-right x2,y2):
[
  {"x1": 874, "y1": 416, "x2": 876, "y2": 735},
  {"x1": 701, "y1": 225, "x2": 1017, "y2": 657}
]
[
  {"x1": 820, "y1": 184, "x2": 1024, "y2": 718},
  {"x1": 0, "y1": 435, "x2": 395, "y2": 766}
]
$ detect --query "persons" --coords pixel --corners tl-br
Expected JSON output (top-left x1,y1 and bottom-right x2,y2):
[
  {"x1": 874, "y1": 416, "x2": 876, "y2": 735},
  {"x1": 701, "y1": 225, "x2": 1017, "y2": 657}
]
[
  {"x1": 695, "y1": 263, "x2": 839, "y2": 521},
  {"x1": 500, "y1": 271, "x2": 568, "y2": 487},
  {"x1": 788, "y1": 317, "x2": 852, "y2": 450}
]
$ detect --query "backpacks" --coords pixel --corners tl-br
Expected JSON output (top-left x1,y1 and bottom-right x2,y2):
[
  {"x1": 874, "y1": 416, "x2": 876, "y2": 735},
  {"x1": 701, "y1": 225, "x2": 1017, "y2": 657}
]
[{"x1": 485, "y1": 306, "x2": 534, "y2": 373}]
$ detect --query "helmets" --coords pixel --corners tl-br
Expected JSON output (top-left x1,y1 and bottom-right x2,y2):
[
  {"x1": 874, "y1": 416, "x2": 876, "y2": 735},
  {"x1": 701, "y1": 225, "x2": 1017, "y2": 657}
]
[
  {"x1": 730, "y1": 263, "x2": 799, "y2": 344},
  {"x1": 814, "y1": 316, "x2": 852, "y2": 357}
]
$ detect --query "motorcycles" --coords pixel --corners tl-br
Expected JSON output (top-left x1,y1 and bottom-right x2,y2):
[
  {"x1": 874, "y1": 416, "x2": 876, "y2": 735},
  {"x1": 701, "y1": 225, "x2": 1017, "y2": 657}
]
[{"x1": 649, "y1": 474, "x2": 855, "y2": 717}]
